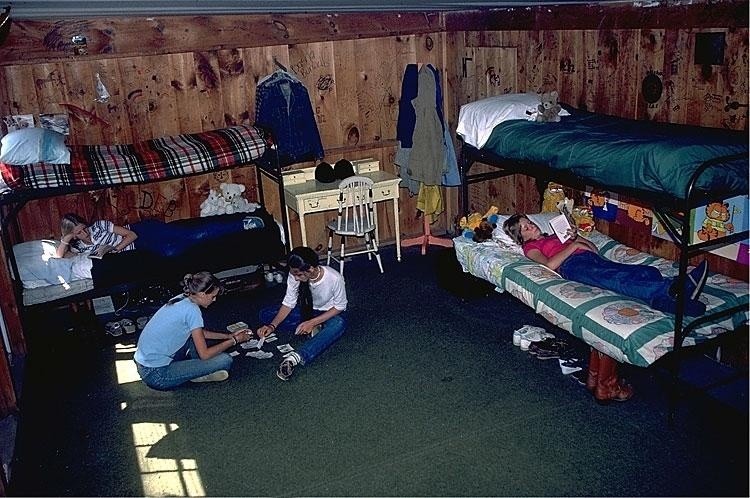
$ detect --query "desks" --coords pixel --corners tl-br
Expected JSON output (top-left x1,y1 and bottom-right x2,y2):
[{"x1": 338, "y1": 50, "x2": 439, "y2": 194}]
[{"x1": 281, "y1": 157, "x2": 404, "y2": 264}]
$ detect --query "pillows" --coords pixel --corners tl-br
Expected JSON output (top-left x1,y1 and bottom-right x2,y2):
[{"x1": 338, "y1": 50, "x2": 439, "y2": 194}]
[
  {"x1": 492, "y1": 205, "x2": 562, "y2": 253},
  {"x1": 457, "y1": 91, "x2": 571, "y2": 151},
  {"x1": 0, "y1": 125, "x2": 70, "y2": 168},
  {"x1": 14, "y1": 236, "x2": 75, "y2": 286}
]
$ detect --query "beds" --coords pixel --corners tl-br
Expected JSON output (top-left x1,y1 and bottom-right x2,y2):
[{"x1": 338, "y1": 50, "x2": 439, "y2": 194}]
[
  {"x1": 455, "y1": 90, "x2": 748, "y2": 429},
  {"x1": 1, "y1": 124, "x2": 293, "y2": 333}
]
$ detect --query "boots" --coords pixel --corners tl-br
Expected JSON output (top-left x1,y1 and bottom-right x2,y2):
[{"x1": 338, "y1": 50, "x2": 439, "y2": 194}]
[
  {"x1": 586, "y1": 345, "x2": 599, "y2": 390},
  {"x1": 594, "y1": 350, "x2": 635, "y2": 403}
]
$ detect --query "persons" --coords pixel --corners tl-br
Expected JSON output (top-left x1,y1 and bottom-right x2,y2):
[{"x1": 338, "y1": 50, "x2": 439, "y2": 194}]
[
  {"x1": 131, "y1": 268, "x2": 256, "y2": 392},
  {"x1": 256, "y1": 244, "x2": 351, "y2": 384},
  {"x1": 54, "y1": 209, "x2": 267, "y2": 259},
  {"x1": 502, "y1": 211, "x2": 712, "y2": 318}
]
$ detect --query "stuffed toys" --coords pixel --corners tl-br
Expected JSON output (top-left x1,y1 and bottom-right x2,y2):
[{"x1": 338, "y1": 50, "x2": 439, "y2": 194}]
[
  {"x1": 536, "y1": 90, "x2": 564, "y2": 125},
  {"x1": 539, "y1": 181, "x2": 597, "y2": 233},
  {"x1": 452, "y1": 202, "x2": 500, "y2": 243},
  {"x1": 524, "y1": 108, "x2": 540, "y2": 123},
  {"x1": 198, "y1": 181, "x2": 262, "y2": 217},
  {"x1": 535, "y1": 73, "x2": 559, "y2": 94}
]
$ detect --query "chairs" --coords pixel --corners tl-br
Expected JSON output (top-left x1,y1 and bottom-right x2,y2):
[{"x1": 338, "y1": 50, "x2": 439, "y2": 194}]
[{"x1": 323, "y1": 175, "x2": 385, "y2": 277}]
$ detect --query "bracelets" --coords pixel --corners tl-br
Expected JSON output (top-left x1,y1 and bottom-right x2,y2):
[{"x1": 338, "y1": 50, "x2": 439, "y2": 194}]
[
  {"x1": 268, "y1": 322, "x2": 278, "y2": 329},
  {"x1": 61, "y1": 238, "x2": 70, "y2": 245},
  {"x1": 231, "y1": 335, "x2": 240, "y2": 345}
]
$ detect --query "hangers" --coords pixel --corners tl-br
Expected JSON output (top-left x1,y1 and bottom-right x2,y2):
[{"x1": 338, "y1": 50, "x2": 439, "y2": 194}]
[{"x1": 257, "y1": 64, "x2": 309, "y2": 94}]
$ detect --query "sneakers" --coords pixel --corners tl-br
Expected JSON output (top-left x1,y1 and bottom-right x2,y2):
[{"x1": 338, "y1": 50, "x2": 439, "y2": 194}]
[
  {"x1": 241, "y1": 216, "x2": 266, "y2": 230},
  {"x1": 674, "y1": 259, "x2": 711, "y2": 301},
  {"x1": 306, "y1": 321, "x2": 324, "y2": 339},
  {"x1": 264, "y1": 270, "x2": 285, "y2": 284},
  {"x1": 104, "y1": 316, "x2": 148, "y2": 337},
  {"x1": 506, "y1": 324, "x2": 576, "y2": 360},
  {"x1": 276, "y1": 356, "x2": 297, "y2": 381},
  {"x1": 571, "y1": 369, "x2": 587, "y2": 386},
  {"x1": 652, "y1": 296, "x2": 706, "y2": 316},
  {"x1": 188, "y1": 370, "x2": 230, "y2": 384}
]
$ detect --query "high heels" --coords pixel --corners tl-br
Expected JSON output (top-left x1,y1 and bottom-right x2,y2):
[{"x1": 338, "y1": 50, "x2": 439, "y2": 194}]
[{"x1": 556, "y1": 347, "x2": 586, "y2": 376}]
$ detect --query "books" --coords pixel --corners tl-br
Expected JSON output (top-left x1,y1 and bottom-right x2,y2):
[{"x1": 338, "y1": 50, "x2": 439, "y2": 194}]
[
  {"x1": 547, "y1": 200, "x2": 577, "y2": 245},
  {"x1": 87, "y1": 243, "x2": 113, "y2": 260},
  {"x1": 226, "y1": 320, "x2": 249, "y2": 333}
]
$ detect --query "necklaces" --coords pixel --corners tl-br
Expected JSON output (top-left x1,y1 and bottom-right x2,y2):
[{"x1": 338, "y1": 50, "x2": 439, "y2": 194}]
[{"x1": 308, "y1": 266, "x2": 321, "y2": 281}]
